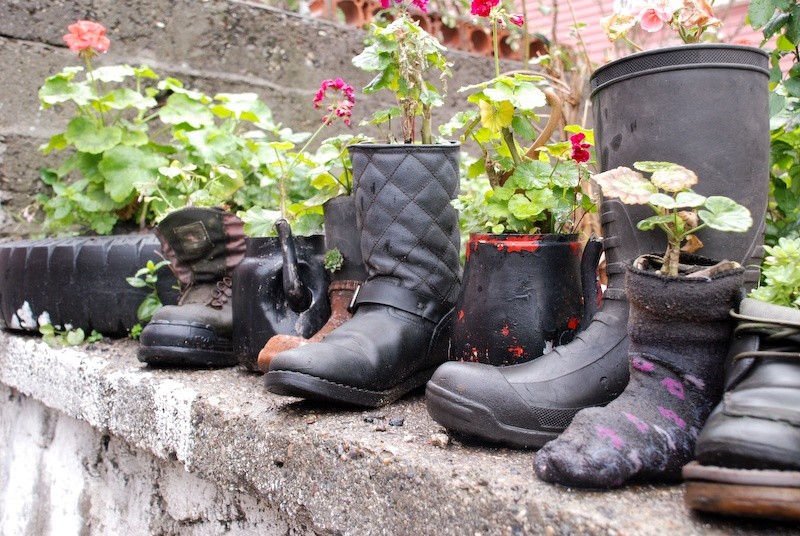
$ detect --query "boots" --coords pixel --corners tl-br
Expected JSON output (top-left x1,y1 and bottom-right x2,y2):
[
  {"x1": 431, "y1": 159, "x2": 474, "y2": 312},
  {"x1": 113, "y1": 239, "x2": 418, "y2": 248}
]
[
  {"x1": 257, "y1": 195, "x2": 369, "y2": 374},
  {"x1": 137, "y1": 206, "x2": 247, "y2": 368},
  {"x1": 265, "y1": 139, "x2": 463, "y2": 406},
  {"x1": 425, "y1": 43, "x2": 770, "y2": 448}
]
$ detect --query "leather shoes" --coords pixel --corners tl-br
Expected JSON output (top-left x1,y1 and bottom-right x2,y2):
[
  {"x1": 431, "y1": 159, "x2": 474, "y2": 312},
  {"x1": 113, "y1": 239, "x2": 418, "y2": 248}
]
[{"x1": 681, "y1": 298, "x2": 800, "y2": 517}]
